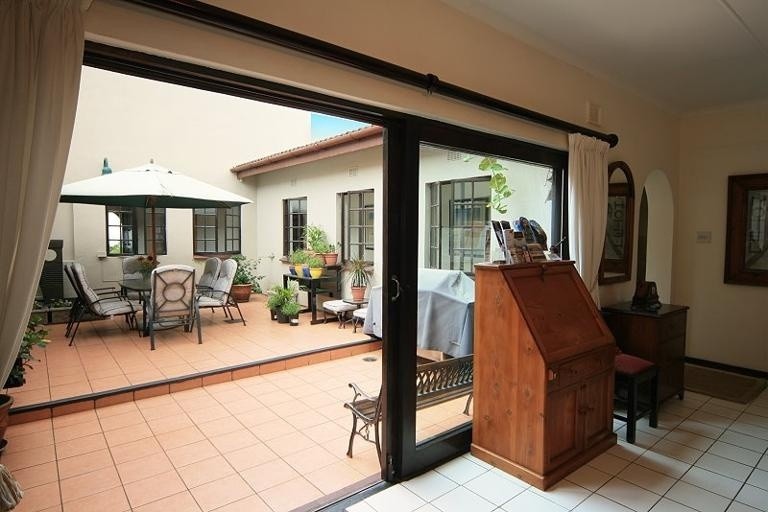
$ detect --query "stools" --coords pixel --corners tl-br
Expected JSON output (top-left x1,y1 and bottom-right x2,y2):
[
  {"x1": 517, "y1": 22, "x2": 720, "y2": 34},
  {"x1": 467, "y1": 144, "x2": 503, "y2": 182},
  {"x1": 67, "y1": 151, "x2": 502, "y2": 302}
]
[
  {"x1": 350, "y1": 308, "x2": 367, "y2": 333},
  {"x1": 319, "y1": 296, "x2": 364, "y2": 329},
  {"x1": 611, "y1": 352, "x2": 662, "y2": 444}
]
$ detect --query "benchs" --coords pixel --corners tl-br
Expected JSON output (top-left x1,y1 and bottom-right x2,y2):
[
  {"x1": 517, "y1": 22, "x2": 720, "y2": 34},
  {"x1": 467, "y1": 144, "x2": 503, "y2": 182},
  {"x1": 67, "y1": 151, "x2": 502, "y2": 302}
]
[{"x1": 336, "y1": 347, "x2": 476, "y2": 464}]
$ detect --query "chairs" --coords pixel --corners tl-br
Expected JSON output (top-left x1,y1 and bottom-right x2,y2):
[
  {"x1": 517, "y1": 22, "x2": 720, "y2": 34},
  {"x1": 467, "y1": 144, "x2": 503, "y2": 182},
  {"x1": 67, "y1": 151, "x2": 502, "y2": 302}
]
[{"x1": 60, "y1": 249, "x2": 247, "y2": 354}]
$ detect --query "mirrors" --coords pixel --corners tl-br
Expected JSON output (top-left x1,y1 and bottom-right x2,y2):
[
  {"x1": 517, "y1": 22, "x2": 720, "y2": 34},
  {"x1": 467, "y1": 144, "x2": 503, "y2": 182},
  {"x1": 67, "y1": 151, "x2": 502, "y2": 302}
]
[{"x1": 598, "y1": 159, "x2": 636, "y2": 286}]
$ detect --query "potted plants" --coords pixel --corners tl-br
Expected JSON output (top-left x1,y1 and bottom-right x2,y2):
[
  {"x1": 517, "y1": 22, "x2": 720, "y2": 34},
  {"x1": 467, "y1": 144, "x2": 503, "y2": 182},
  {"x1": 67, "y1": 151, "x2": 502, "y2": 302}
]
[
  {"x1": 302, "y1": 222, "x2": 344, "y2": 268},
  {"x1": 335, "y1": 250, "x2": 375, "y2": 302},
  {"x1": 284, "y1": 249, "x2": 326, "y2": 279},
  {"x1": 223, "y1": 252, "x2": 266, "y2": 304},
  {"x1": 27, "y1": 300, "x2": 50, "y2": 326},
  {"x1": 265, "y1": 283, "x2": 305, "y2": 327},
  {"x1": 0, "y1": 309, "x2": 55, "y2": 456},
  {"x1": 46, "y1": 297, "x2": 107, "y2": 325}
]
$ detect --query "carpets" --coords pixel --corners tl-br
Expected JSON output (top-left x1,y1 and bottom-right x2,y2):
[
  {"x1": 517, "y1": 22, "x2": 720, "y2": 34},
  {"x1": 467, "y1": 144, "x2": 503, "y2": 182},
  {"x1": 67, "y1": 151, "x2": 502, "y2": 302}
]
[{"x1": 680, "y1": 359, "x2": 767, "y2": 406}]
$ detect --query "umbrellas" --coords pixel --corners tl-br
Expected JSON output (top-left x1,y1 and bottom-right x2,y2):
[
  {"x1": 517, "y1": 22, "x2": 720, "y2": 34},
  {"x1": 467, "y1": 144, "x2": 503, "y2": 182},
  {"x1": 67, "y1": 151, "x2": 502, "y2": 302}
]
[{"x1": 59, "y1": 157, "x2": 255, "y2": 266}]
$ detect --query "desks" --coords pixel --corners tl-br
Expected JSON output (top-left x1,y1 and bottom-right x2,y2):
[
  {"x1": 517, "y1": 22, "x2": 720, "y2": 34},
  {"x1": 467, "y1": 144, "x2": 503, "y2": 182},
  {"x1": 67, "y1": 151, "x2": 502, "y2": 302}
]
[{"x1": 337, "y1": 297, "x2": 371, "y2": 334}]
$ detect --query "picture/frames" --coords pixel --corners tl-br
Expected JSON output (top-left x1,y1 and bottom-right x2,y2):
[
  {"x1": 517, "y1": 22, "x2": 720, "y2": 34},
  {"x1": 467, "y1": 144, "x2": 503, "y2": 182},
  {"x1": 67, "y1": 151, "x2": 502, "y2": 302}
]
[
  {"x1": 719, "y1": 169, "x2": 767, "y2": 291},
  {"x1": 604, "y1": 182, "x2": 630, "y2": 274}
]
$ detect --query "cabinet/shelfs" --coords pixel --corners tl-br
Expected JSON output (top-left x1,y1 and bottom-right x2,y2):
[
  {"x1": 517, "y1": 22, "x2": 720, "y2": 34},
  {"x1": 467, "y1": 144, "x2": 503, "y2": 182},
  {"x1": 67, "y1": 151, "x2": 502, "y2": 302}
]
[
  {"x1": 598, "y1": 293, "x2": 692, "y2": 418},
  {"x1": 462, "y1": 255, "x2": 620, "y2": 492},
  {"x1": 281, "y1": 262, "x2": 344, "y2": 326}
]
